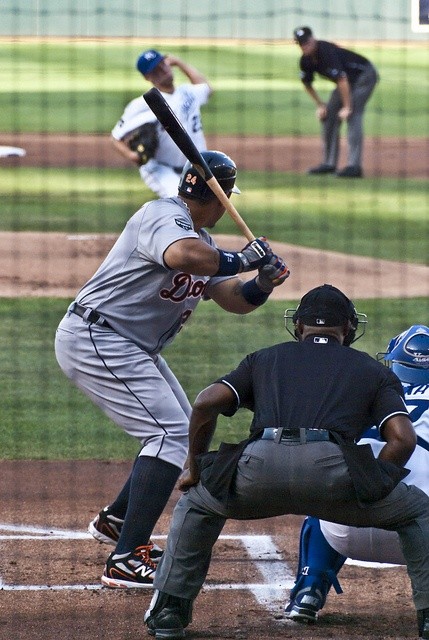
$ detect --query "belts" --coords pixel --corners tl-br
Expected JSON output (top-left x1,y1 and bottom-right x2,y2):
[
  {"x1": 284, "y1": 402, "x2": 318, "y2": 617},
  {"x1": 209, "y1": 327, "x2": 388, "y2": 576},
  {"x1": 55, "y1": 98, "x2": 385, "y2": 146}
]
[
  {"x1": 261, "y1": 428, "x2": 329, "y2": 442},
  {"x1": 70, "y1": 303, "x2": 113, "y2": 328}
]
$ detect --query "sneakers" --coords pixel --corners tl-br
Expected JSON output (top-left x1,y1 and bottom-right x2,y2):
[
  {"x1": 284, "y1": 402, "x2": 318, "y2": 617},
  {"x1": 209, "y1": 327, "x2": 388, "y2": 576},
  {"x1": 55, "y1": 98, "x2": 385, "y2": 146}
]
[
  {"x1": 101, "y1": 545, "x2": 157, "y2": 589},
  {"x1": 88, "y1": 505, "x2": 164, "y2": 561},
  {"x1": 309, "y1": 164, "x2": 336, "y2": 174},
  {"x1": 338, "y1": 166, "x2": 362, "y2": 177},
  {"x1": 147, "y1": 608, "x2": 184, "y2": 640},
  {"x1": 285, "y1": 600, "x2": 318, "y2": 626}
]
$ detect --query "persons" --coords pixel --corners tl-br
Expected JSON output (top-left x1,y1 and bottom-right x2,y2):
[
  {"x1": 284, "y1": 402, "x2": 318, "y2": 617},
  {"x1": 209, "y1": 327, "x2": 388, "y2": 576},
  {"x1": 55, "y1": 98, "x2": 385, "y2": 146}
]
[
  {"x1": 294, "y1": 26, "x2": 379, "y2": 177},
  {"x1": 145, "y1": 284, "x2": 429, "y2": 639},
  {"x1": 284, "y1": 324, "x2": 428, "y2": 620},
  {"x1": 54, "y1": 150, "x2": 289, "y2": 586},
  {"x1": 110, "y1": 49, "x2": 213, "y2": 201}
]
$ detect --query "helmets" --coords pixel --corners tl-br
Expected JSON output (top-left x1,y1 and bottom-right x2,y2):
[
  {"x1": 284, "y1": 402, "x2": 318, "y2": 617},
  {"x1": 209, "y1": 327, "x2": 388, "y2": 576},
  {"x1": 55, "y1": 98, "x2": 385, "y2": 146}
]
[
  {"x1": 376, "y1": 323, "x2": 429, "y2": 385},
  {"x1": 284, "y1": 284, "x2": 368, "y2": 346},
  {"x1": 178, "y1": 151, "x2": 242, "y2": 202}
]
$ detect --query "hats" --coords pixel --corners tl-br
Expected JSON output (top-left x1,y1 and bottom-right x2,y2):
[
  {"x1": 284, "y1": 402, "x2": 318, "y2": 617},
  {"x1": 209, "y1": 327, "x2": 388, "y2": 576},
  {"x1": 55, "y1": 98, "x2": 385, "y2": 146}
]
[
  {"x1": 293, "y1": 26, "x2": 312, "y2": 44},
  {"x1": 136, "y1": 49, "x2": 168, "y2": 76}
]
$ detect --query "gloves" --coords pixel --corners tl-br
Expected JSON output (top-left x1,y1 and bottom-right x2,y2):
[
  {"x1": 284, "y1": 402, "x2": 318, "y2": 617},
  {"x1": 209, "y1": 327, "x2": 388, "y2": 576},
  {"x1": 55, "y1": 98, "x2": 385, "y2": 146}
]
[
  {"x1": 258, "y1": 254, "x2": 290, "y2": 286},
  {"x1": 235, "y1": 238, "x2": 273, "y2": 273}
]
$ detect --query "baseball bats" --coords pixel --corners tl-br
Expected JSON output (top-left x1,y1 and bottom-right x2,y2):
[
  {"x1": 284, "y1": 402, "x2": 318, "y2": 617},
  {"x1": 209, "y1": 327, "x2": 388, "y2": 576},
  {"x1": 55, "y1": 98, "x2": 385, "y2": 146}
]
[{"x1": 142, "y1": 85, "x2": 257, "y2": 243}]
